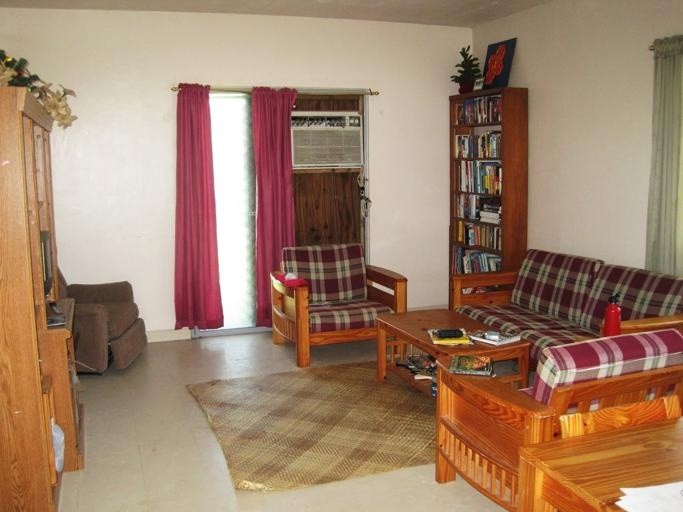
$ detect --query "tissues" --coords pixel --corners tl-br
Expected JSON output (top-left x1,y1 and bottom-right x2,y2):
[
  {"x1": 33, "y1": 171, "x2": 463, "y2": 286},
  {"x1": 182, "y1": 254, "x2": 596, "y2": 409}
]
[{"x1": 273, "y1": 272, "x2": 306, "y2": 286}]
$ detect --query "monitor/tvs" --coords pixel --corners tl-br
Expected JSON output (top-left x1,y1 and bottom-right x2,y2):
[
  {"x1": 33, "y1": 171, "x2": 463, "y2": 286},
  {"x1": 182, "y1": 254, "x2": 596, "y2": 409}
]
[{"x1": 39, "y1": 230, "x2": 52, "y2": 296}]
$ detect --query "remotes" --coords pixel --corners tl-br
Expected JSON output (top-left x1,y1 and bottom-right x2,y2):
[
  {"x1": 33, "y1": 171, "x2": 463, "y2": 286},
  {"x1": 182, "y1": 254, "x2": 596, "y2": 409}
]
[{"x1": 51, "y1": 304, "x2": 63, "y2": 315}]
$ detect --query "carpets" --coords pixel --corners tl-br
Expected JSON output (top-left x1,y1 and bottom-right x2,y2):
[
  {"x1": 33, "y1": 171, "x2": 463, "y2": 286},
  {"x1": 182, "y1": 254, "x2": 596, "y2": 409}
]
[{"x1": 184, "y1": 358, "x2": 440, "y2": 494}]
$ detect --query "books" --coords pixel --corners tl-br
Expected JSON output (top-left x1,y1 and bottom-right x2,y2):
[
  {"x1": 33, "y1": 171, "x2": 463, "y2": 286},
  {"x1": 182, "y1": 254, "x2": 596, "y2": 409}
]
[
  {"x1": 452, "y1": 93, "x2": 502, "y2": 296},
  {"x1": 427, "y1": 327, "x2": 472, "y2": 346},
  {"x1": 449, "y1": 353, "x2": 494, "y2": 378},
  {"x1": 468, "y1": 327, "x2": 521, "y2": 348}
]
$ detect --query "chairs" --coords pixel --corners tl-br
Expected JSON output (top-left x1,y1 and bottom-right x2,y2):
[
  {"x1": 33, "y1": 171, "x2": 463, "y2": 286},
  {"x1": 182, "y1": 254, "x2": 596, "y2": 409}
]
[
  {"x1": 55, "y1": 265, "x2": 148, "y2": 377},
  {"x1": 427, "y1": 332, "x2": 683, "y2": 512},
  {"x1": 270, "y1": 240, "x2": 409, "y2": 369}
]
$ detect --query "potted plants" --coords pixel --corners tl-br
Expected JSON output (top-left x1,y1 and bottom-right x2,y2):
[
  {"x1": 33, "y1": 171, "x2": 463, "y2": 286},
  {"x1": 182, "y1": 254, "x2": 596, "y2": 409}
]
[{"x1": 449, "y1": 45, "x2": 483, "y2": 94}]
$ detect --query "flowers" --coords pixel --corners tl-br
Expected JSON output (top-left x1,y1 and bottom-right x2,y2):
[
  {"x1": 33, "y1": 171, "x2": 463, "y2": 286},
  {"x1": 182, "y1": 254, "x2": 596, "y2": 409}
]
[{"x1": 4, "y1": 52, "x2": 78, "y2": 132}]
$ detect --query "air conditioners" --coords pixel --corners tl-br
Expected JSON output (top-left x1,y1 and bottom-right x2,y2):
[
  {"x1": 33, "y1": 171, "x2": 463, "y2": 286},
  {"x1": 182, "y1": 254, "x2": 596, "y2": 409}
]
[{"x1": 289, "y1": 109, "x2": 364, "y2": 169}]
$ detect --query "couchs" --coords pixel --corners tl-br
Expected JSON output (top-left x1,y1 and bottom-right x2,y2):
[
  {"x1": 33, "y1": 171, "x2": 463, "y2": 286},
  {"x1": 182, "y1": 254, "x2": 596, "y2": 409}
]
[{"x1": 448, "y1": 248, "x2": 682, "y2": 371}]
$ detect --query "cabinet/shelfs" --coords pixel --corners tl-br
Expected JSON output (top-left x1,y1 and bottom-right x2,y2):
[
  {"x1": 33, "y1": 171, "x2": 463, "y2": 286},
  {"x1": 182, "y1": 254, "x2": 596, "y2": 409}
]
[
  {"x1": 446, "y1": 87, "x2": 528, "y2": 308},
  {"x1": 0, "y1": 84, "x2": 83, "y2": 511}
]
